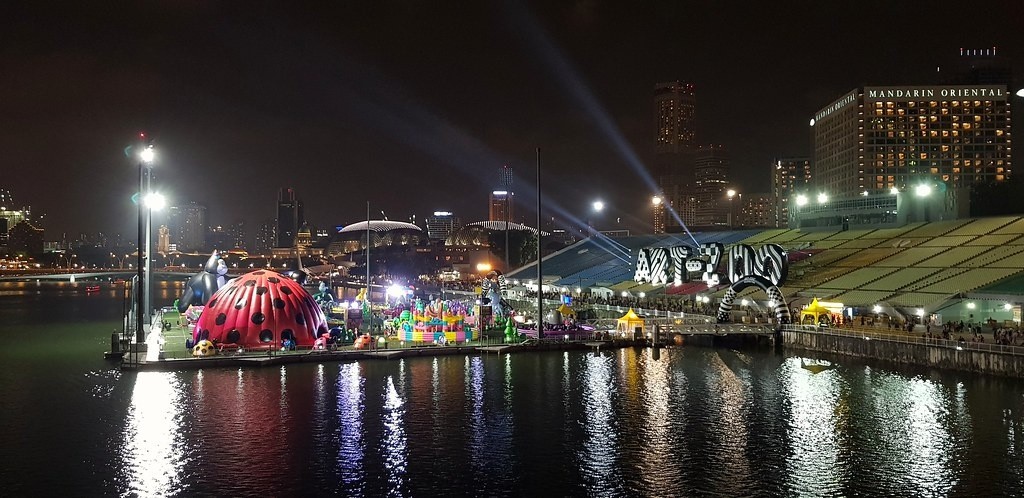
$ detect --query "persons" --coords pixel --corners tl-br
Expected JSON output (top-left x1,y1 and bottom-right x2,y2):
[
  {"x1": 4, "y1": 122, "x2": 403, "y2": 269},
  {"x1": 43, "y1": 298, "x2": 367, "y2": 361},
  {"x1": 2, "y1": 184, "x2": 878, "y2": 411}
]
[{"x1": 419, "y1": 278, "x2": 1024, "y2": 348}]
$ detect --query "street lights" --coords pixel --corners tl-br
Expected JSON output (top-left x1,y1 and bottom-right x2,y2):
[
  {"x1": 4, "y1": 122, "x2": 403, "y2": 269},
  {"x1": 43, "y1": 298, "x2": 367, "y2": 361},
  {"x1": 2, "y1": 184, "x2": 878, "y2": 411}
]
[
  {"x1": 348, "y1": 302, "x2": 360, "y2": 310},
  {"x1": 138, "y1": 146, "x2": 154, "y2": 342},
  {"x1": 723, "y1": 188, "x2": 739, "y2": 204},
  {"x1": 649, "y1": 194, "x2": 663, "y2": 210},
  {"x1": 143, "y1": 189, "x2": 165, "y2": 323},
  {"x1": 591, "y1": 198, "x2": 607, "y2": 213}
]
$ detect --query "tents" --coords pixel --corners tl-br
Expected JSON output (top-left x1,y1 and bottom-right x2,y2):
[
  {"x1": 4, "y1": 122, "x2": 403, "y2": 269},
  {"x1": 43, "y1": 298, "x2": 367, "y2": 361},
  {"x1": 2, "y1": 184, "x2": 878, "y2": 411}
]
[
  {"x1": 618, "y1": 307, "x2": 644, "y2": 338},
  {"x1": 555, "y1": 303, "x2": 575, "y2": 322},
  {"x1": 801, "y1": 296, "x2": 832, "y2": 326}
]
